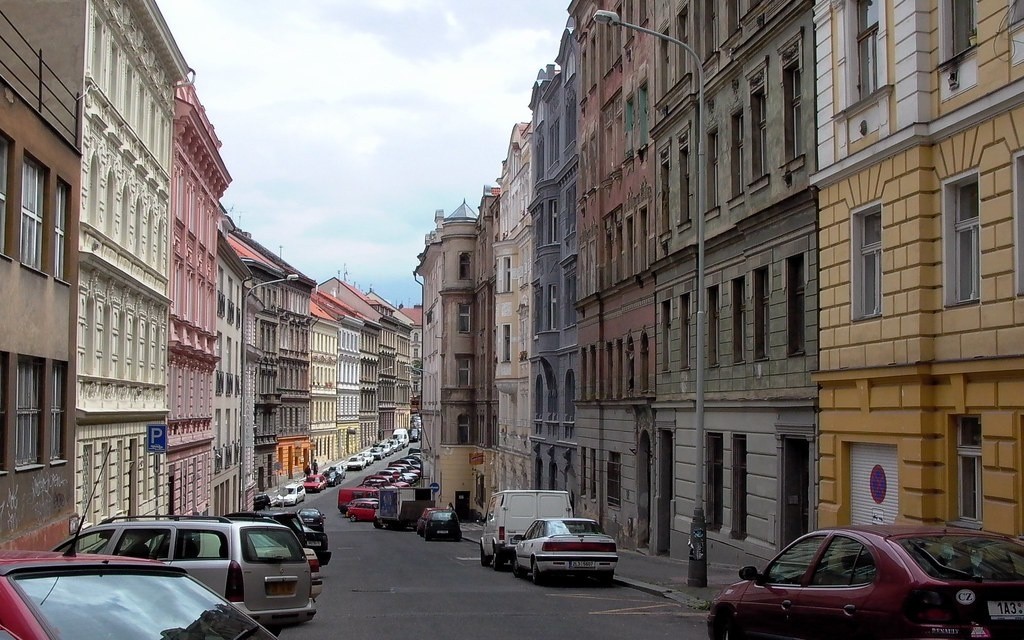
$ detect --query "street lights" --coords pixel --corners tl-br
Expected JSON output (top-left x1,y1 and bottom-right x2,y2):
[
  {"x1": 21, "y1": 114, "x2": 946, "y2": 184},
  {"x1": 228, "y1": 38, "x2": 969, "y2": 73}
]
[
  {"x1": 241, "y1": 274, "x2": 300, "y2": 511},
  {"x1": 594, "y1": 9, "x2": 707, "y2": 586}
]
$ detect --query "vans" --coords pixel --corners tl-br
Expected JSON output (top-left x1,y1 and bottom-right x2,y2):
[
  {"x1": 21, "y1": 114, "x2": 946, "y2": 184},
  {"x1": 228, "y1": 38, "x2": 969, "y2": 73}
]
[
  {"x1": 480, "y1": 490, "x2": 575, "y2": 572},
  {"x1": 393, "y1": 429, "x2": 409, "y2": 447},
  {"x1": 408, "y1": 448, "x2": 421, "y2": 454}
]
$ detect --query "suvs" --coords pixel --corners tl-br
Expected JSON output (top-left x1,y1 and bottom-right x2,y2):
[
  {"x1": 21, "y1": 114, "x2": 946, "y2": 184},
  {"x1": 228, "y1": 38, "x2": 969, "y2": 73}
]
[
  {"x1": 298, "y1": 507, "x2": 327, "y2": 528},
  {"x1": 226, "y1": 511, "x2": 323, "y2": 599},
  {"x1": 51, "y1": 516, "x2": 316, "y2": 637},
  {"x1": 254, "y1": 493, "x2": 272, "y2": 510},
  {"x1": 277, "y1": 484, "x2": 306, "y2": 505}
]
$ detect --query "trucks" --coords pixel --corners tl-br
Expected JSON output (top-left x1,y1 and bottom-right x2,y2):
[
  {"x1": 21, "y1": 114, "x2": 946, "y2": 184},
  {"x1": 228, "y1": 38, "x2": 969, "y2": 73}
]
[
  {"x1": 339, "y1": 489, "x2": 382, "y2": 511},
  {"x1": 375, "y1": 487, "x2": 436, "y2": 530}
]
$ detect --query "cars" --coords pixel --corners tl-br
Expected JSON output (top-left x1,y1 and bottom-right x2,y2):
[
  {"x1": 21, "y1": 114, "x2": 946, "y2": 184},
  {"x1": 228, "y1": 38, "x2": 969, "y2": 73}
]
[
  {"x1": 0, "y1": 549, "x2": 276, "y2": 640},
  {"x1": 410, "y1": 415, "x2": 422, "y2": 441},
  {"x1": 302, "y1": 439, "x2": 403, "y2": 493},
  {"x1": 345, "y1": 497, "x2": 378, "y2": 521},
  {"x1": 417, "y1": 508, "x2": 462, "y2": 542},
  {"x1": 706, "y1": 524, "x2": 1024, "y2": 640},
  {"x1": 356, "y1": 453, "x2": 423, "y2": 488},
  {"x1": 512, "y1": 518, "x2": 619, "y2": 586}
]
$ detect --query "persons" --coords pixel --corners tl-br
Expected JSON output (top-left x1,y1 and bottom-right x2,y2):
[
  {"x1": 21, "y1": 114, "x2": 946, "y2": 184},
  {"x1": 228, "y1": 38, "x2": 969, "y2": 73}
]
[
  {"x1": 312, "y1": 459, "x2": 318, "y2": 475},
  {"x1": 448, "y1": 503, "x2": 454, "y2": 512},
  {"x1": 305, "y1": 465, "x2": 311, "y2": 476}
]
